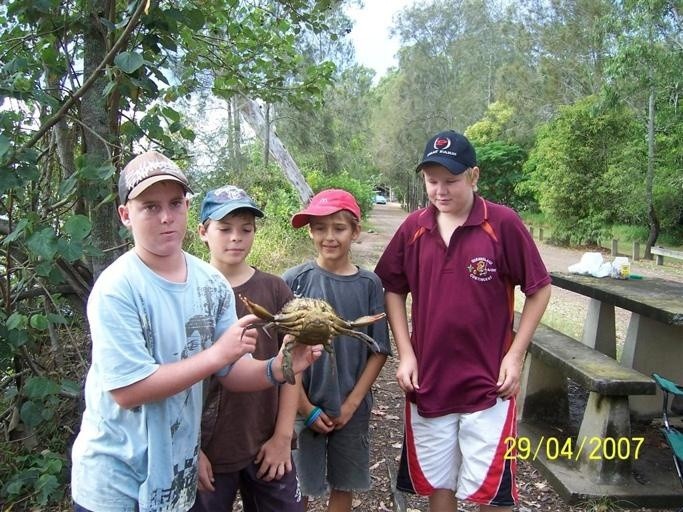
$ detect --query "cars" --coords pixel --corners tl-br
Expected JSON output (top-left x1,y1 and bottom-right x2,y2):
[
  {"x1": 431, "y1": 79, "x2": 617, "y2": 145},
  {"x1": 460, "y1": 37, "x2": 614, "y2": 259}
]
[{"x1": 376, "y1": 196, "x2": 386, "y2": 204}]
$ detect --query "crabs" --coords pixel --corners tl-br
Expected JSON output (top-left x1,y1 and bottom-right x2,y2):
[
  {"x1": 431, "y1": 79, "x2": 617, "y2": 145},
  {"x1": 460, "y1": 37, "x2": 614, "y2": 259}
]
[{"x1": 239, "y1": 293, "x2": 387, "y2": 385}]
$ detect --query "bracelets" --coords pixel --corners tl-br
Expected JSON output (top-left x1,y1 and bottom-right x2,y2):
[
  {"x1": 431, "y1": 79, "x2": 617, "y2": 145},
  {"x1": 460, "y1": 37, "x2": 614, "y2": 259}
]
[
  {"x1": 265, "y1": 357, "x2": 286, "y2": 386},
  {"x1": 303, "y1": 406, "x2": 321, "y2": 428}
]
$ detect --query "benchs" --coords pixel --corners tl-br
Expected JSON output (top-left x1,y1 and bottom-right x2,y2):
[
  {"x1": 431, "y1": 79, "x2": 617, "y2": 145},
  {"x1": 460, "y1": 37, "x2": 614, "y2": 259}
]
[{"x1": 510, "y1": 310, "x2": 657, "y2": 486}]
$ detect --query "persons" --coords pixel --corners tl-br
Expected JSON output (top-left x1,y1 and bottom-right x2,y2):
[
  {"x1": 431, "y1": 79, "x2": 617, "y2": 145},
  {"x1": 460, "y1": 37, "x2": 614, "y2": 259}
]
[
  {"x1": 280, "y1": 189, "x2": 395, "y2": 512},
  {"x1": 184, "y1": 186, "x2": 308, "y2": 511},
  {"x1": 374, "y1": 132, "x2": 553, "y2": 511},
  {"x1": 62, "y1": 149, "x2": 324, "y2": 511}
]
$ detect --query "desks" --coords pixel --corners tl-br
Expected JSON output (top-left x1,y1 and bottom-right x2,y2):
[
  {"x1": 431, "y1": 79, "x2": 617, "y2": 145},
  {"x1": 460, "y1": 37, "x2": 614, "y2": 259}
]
[{"x1": 547, "y1": 271, "x2": 683, "y2": 421}]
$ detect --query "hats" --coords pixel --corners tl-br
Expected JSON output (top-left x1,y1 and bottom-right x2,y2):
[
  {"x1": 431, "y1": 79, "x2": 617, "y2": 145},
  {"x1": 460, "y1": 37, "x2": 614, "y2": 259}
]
[
  {"x1": 414, "y1": 130, "x2": 478, "y2": 176},
  {"x1": 290, "y1": 187, "x2": 362, "y2": 229},
  {"x1": 199, "y1": 185, "x2": 264, "y2": 225},
  {"x1": 116, "y1": 149, "x2": 195, "y2": 206}
]
